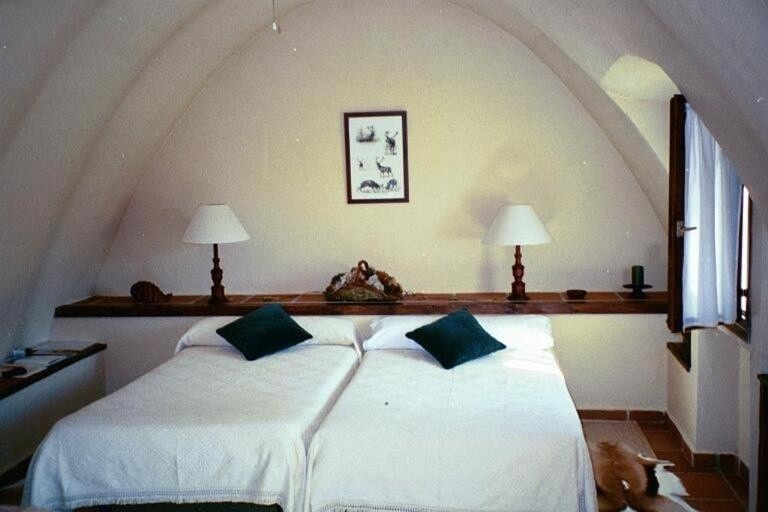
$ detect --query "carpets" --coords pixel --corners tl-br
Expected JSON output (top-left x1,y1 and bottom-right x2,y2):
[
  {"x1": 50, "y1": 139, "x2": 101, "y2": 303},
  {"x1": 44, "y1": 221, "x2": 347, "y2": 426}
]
[{"x1": 580, "y1": 415, "x2": 696, "y2": 512}]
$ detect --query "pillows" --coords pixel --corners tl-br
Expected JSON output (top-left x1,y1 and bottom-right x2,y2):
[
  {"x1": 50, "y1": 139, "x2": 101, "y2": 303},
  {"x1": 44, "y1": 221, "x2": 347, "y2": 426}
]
[
  {"x1": 361, "y1": 308, "x2": 553, "y2": 371},
  {"x1": 182, "y1": 302, "x2": 355, "y2": 361}
]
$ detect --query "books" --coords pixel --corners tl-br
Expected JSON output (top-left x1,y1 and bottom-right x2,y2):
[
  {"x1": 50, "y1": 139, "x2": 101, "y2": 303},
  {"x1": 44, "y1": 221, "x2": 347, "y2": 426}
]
[
  {"x1": 30, "y1": 339, "x2": 96, "y2": 352},
  {"x1": 10, "y1": 355, "x2": 70, "y2": 368},
  {"x1": 0, "y1": 361, "x2": 48, "y2": 380}
]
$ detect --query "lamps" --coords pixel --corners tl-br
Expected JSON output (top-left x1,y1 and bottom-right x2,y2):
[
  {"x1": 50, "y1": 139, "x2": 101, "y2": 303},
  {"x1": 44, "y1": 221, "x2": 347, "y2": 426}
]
[
  {"x1": 182, "y1": 203, "x2": 251, "y2": 303},
  {"x1": 484, "y1": 204, "x2": 553, "y2": 301}
]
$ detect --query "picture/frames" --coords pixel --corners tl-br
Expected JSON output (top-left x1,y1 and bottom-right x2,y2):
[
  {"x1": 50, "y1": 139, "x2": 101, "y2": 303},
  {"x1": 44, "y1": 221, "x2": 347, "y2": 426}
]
[{"x1": 344, "y1": 109, "x2": 411, "y2": 204}]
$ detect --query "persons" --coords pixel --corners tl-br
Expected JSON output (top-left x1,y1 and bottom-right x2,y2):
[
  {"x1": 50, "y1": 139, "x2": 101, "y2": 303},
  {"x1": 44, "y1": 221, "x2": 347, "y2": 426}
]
[{"x1": 0, "y1": 363, "x2": 28, "y2": 379}]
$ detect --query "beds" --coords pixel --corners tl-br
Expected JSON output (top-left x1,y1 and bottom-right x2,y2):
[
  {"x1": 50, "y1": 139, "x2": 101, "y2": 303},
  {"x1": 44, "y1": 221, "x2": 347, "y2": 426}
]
[
  {"x1": 306, "y1": 349, "x2": 586, "y2": 509},
  {"x1": 27, "y1": 349, "x2": 363, "y2": 510}
]
